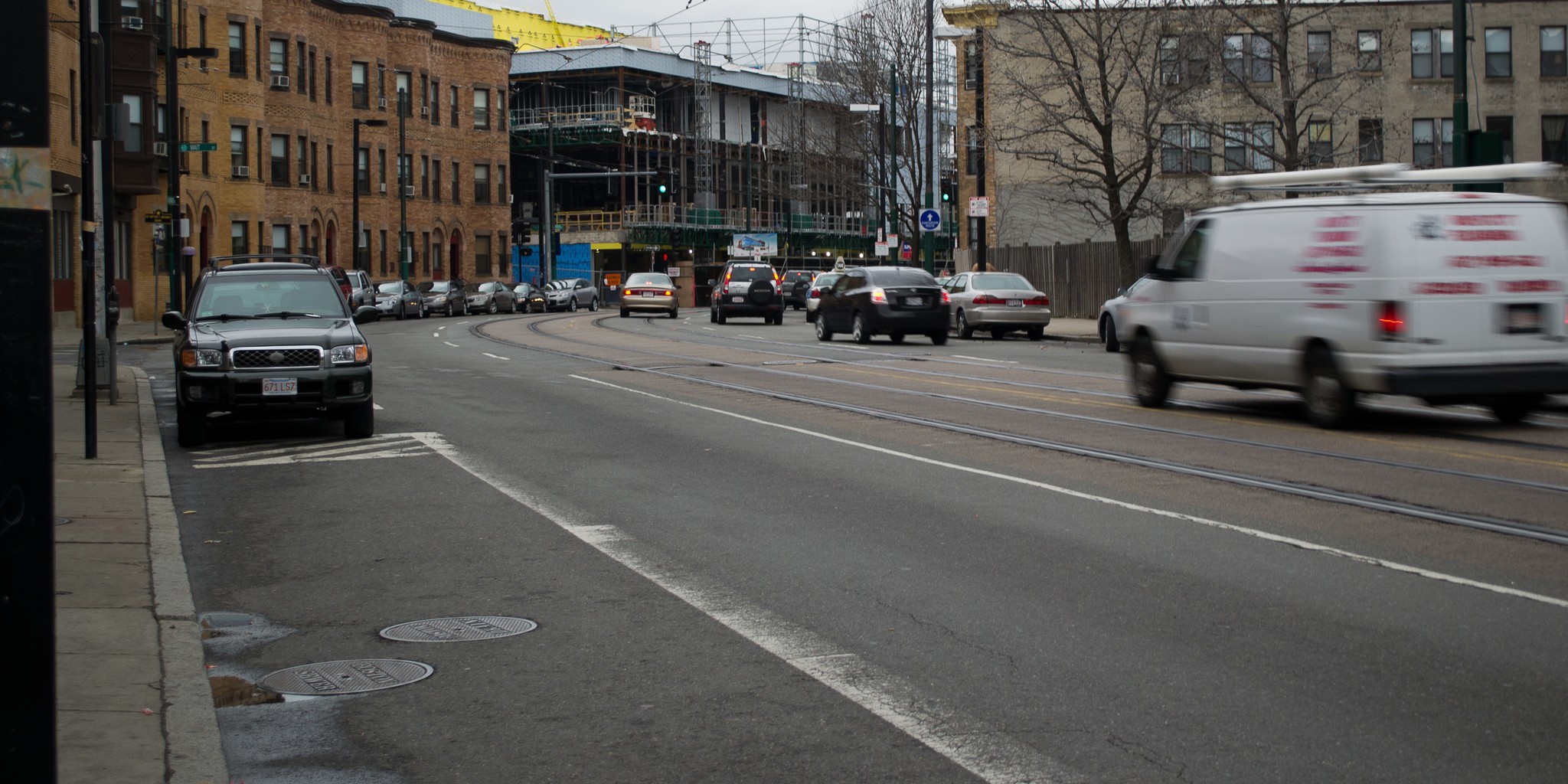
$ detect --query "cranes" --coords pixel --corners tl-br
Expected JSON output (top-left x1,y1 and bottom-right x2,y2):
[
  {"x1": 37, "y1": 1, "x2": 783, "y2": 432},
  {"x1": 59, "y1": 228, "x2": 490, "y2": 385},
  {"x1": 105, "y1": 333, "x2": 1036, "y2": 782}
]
[
  {"x1": 693, "y1": 39, "x2": 716, "y2": 211},
  {"x1": 787, "y1": 60, "x2": 810, "y2": 215}
]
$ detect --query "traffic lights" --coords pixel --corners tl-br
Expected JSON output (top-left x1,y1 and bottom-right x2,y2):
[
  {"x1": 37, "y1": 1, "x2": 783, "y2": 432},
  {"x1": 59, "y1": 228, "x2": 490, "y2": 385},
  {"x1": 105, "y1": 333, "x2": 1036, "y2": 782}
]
[
  {"x1": 511, "y1": 219, "x2": 531, "y2": 256},
  {"x1": 940, "y1": 178, "x2": 952, "y2": 202},
  {"x1": 655, "y1": 167, "x2": 672, "y2": 197}
]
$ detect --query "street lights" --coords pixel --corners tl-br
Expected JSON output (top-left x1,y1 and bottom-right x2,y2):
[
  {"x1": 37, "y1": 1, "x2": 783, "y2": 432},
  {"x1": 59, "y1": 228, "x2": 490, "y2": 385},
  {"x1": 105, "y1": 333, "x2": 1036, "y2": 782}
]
[
  {"x1": 351, "y1": 117, "x2": 386, "y2": 270},
  {"x1": 171, "y1": 42, "x2": 218, "y2": 317}
]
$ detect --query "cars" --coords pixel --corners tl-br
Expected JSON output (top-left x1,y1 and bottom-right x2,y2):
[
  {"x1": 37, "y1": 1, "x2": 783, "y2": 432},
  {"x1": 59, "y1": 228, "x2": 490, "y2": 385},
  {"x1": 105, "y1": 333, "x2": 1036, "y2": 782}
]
[
  {"x1": 619, "y1": 272, "x2": 682, "y2": 318},
  {"x1": 462, "y1": 280, "x2": 518, "y2": 315},
  {"x1": 506, "y1": 282, "x2": 548, "y2": 313},
  {"x1": 806, "y1": 256, "x2": 847, "y2": 325},
  {"x1": 367, "y1": 280, "x2": 424, "y2": 321},
  {"x1": 815, "y1": 265, "x2": 951, "y2": 345},
  {"x1": 932, "y1": 270, "x2": 954, "y2": 287},
  {"x1": 415, "y1": 280, "x2": 467, "y2": 317},
  {"x1": 940, "y1": 271, "x2": 1051, "y2": 338},
  {"x1": 1097, "y1": 272, "x2": 1154, "y2": 351}
]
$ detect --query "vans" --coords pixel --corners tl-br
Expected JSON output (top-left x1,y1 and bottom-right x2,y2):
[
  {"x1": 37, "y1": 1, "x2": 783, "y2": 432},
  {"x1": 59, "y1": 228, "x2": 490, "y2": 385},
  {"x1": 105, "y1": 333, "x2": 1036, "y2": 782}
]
[{"x1": 1121, "y1": 160, "x2": 1568, "y2": 429}]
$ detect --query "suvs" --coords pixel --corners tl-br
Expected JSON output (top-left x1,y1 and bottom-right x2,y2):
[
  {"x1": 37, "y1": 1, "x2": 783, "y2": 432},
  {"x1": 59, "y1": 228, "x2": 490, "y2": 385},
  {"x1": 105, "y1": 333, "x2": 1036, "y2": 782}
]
[
  {"x1": 541, "y1": 277, "x2": 599, "y2": 312},
  {"x1": 708, "y1": 259, "x2": 784, "y2": 325},
  {"x1": 781, "y1": 269, "x2": 821, "y2": 311},
  {"x1": 345, "y1": 267, "x2": 374, "y2": 324},
  {"x1": 317, "y1": 263, "x2": 354, "y2": 322},
  {"x1": 161, "y1": 254, "x2": 379, "y2": 446}
]
